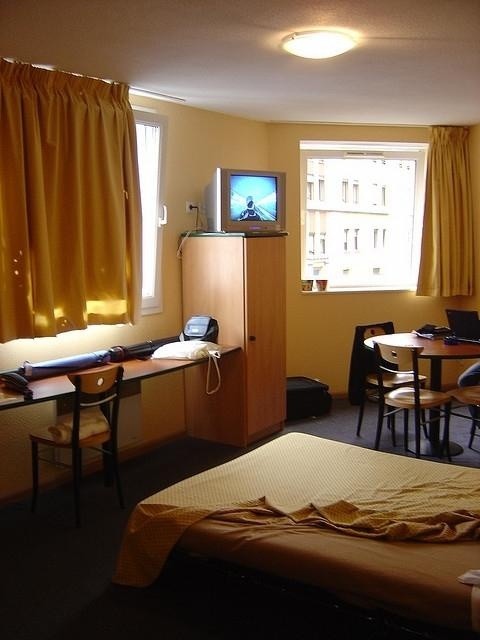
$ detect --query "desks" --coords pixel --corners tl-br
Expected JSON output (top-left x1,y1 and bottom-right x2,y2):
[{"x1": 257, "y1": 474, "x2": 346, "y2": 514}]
[
  {"x1": 363, "y1": 331, "x2": 480, "y2": 456},
  {"x1": 0, "y1": 344, "x2": 241, "y2": 411}
]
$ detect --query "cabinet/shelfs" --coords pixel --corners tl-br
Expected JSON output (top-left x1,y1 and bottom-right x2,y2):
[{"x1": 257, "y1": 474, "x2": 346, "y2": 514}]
[{"x1": 181, "y1": 231, "x2": 289, "y2": 450}]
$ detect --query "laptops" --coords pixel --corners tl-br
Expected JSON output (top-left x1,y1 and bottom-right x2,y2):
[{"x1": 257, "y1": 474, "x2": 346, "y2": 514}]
[{"x1": 445, "y1": 309, "x2": 480, "y2": 343}]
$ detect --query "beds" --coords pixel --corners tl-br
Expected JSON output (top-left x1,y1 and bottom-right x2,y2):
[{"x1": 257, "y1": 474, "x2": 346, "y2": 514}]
[{"x1": 136, "y1": 430, "x2": 480, "y2": 640}]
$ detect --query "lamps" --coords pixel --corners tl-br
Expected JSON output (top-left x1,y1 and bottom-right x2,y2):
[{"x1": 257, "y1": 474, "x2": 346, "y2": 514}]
[{"x1": 279, "y1": 28, "x2": 358, "y2": 60}]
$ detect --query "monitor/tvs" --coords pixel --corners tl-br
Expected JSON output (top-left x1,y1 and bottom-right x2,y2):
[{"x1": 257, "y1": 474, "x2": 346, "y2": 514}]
[{"x1": 205, "y1": 167, "x2": 286, "y2": 233}]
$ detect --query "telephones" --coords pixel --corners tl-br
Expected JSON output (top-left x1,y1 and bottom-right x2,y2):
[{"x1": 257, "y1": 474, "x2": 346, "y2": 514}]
[{"x1": 3, "y1": 372, "x2": 29, "y2": 392}]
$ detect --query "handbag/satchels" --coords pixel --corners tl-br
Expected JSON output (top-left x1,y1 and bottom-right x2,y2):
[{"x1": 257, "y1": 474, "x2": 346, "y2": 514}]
[{"x1": 185, "y1": 315, "x2": 219, "y2": 345}]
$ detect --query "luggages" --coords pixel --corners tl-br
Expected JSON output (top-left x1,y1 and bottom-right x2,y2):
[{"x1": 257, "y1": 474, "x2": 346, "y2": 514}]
[{"x1": 286, "y1": 372, "x2": 331, "y2": 419}]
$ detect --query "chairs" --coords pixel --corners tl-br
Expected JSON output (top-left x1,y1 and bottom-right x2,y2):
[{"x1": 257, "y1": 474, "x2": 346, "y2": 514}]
[
  {"x1": 447, "y1": 385, "x2": 480, "y2": 449},
  {"x1": 354, "y1": 321, "x2": 430, "y2": 441},
  {"x1": 28, "y1": 364, "x2": 140, "y2": 519},
  {"x1": 373, "y1": 342, "x2": 453, "y2": 464}
]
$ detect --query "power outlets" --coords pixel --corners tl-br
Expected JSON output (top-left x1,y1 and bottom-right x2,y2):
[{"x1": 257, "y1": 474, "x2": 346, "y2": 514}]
[{"x1": 186, "y1": 200, "x2": 196, "y2": 213}]
